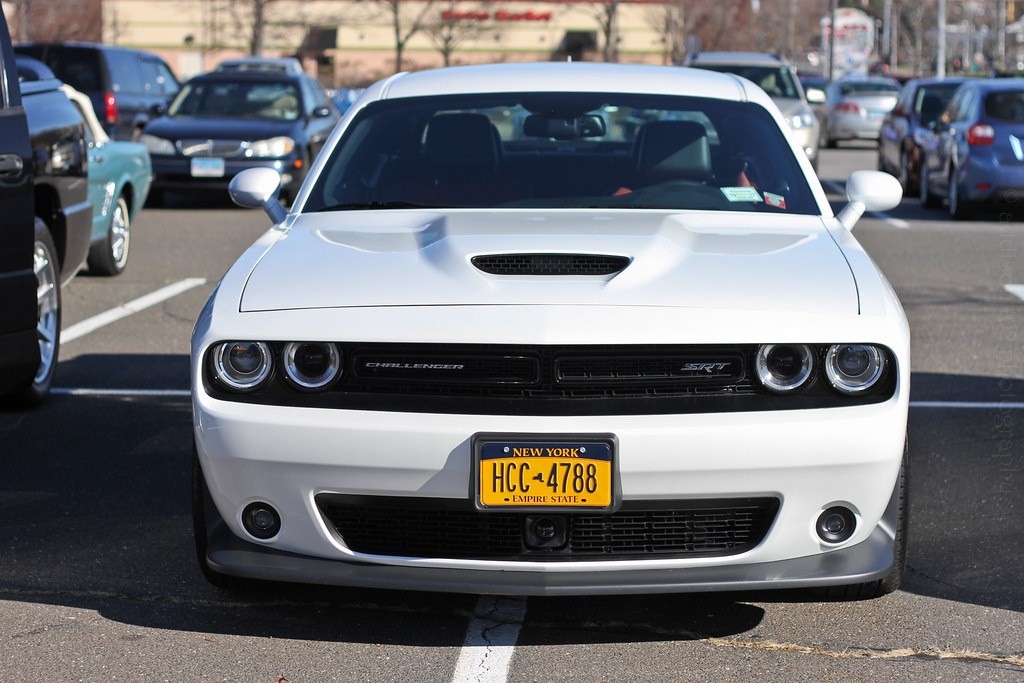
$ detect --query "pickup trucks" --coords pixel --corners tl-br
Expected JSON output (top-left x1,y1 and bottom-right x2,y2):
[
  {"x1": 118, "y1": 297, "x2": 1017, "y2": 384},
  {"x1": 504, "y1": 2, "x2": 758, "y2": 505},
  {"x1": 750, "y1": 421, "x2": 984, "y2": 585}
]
[{"x1": 0, "y1": 5, "x2": 95, "y2": 411}]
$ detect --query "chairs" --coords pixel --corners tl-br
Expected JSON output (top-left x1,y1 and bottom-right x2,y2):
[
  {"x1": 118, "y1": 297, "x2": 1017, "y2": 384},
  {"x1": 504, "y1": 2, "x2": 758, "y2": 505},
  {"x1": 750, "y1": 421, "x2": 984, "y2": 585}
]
[
  {"x1": 616, "y1": 119, "x2": 752, "y2": 200},
  {"x1": 272, "y1": 96, "x2": 299, "y2": 116},
  {"x1": 393, "y1": 114, "x2": 517, "y2": 201}
]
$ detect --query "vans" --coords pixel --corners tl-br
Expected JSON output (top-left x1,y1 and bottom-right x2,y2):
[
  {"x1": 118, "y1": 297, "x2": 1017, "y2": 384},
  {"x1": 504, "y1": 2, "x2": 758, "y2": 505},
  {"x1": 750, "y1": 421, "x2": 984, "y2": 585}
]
[{"x1": 13, "y1": 40, "x2": 182, "y2": 140}]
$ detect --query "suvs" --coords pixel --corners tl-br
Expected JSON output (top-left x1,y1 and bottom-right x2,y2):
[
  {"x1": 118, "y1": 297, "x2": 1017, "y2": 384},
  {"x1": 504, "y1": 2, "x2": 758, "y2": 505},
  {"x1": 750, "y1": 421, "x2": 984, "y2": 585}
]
[{"x1": 679, "y1": 50, "x2": 823, "y2": 173}]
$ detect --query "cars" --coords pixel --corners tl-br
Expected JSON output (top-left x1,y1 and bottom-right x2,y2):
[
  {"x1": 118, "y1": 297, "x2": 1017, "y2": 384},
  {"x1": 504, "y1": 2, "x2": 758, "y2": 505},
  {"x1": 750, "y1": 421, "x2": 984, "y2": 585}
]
[
  {"x1": 191, "y1": 59, "x2": 911, "y2": 598},
  {"x1": 824, "y1": 75, "x2": 907, "y2": 149},
  {"x1": 879, "y1": 77, "x2": 974, "y2": 198},
  {"x1": 137, "y1": 68, "x2": 340, "y2": 208},
  {"x1": 217, "y1": 59, "x2": 304, "y2": 109},
  {"x1": 60, "y1": 85, "x2": 155, "y2": 279},
  {"x1": 921, "y1": 78, "x2": 1024, "y2": 218}
]
